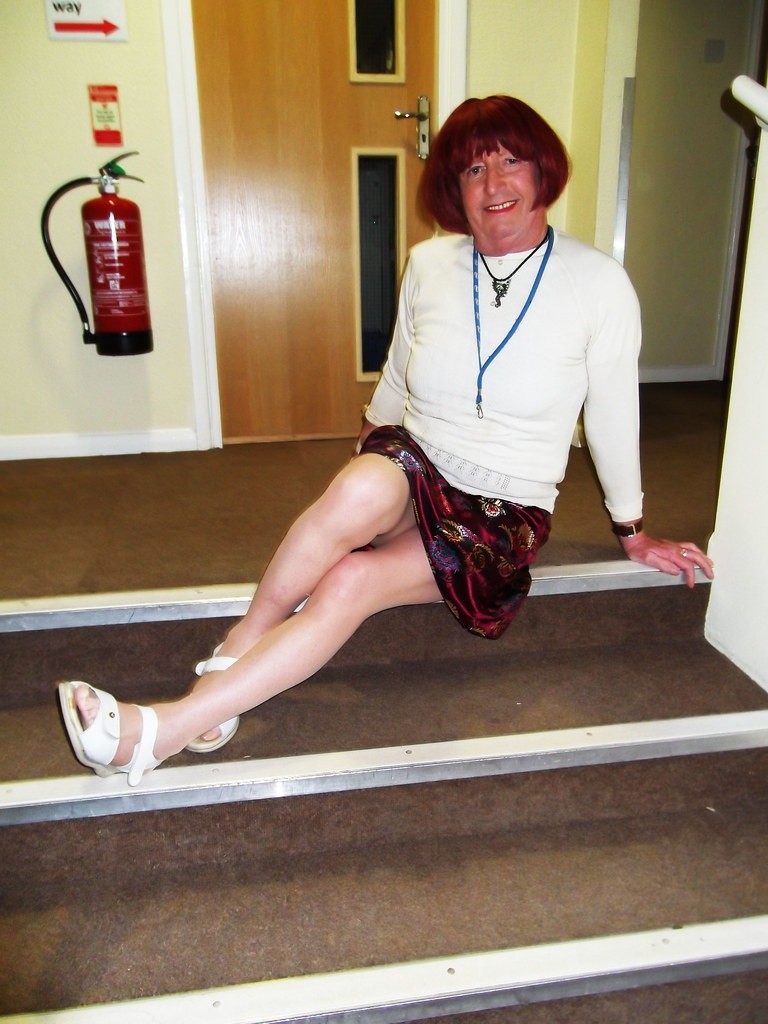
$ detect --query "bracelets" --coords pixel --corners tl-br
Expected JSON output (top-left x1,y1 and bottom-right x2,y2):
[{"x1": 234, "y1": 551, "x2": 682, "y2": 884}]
[
  {"x1": 356, "y1": 439, "x2": 362, "y2": 454},
  {"x1": 613, "y1": 521, "x2": 643, "y2": 537}
]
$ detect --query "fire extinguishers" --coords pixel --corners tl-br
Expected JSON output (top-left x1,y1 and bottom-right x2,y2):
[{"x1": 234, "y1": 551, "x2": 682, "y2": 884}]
[{"x1": 39, "y1": 151, "x2": 155, "y2": 357}]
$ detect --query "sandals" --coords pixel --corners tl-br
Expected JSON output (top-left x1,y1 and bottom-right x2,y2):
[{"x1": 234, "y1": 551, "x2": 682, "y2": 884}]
[
  {"x1": 183, "y1": 640, "x2": 241, "y2": 753},
  {"x1": 58, "y1": 681, "x2": 162, "y2": 787}
]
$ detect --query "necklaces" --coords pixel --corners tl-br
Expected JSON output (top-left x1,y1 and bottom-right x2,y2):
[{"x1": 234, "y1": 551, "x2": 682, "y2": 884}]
[{"x1": 473, "y1": 225, "x2": 554, "y2": 419}]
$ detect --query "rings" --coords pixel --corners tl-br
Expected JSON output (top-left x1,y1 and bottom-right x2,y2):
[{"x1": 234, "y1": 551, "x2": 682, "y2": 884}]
[{"x1": 680, "y1": 548, "x2": 688, "y2": 556}]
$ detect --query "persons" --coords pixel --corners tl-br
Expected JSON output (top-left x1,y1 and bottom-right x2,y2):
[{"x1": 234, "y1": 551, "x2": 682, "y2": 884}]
[{"x1": 58, "y1": 94, "x2": 714, "y2": 787}]
